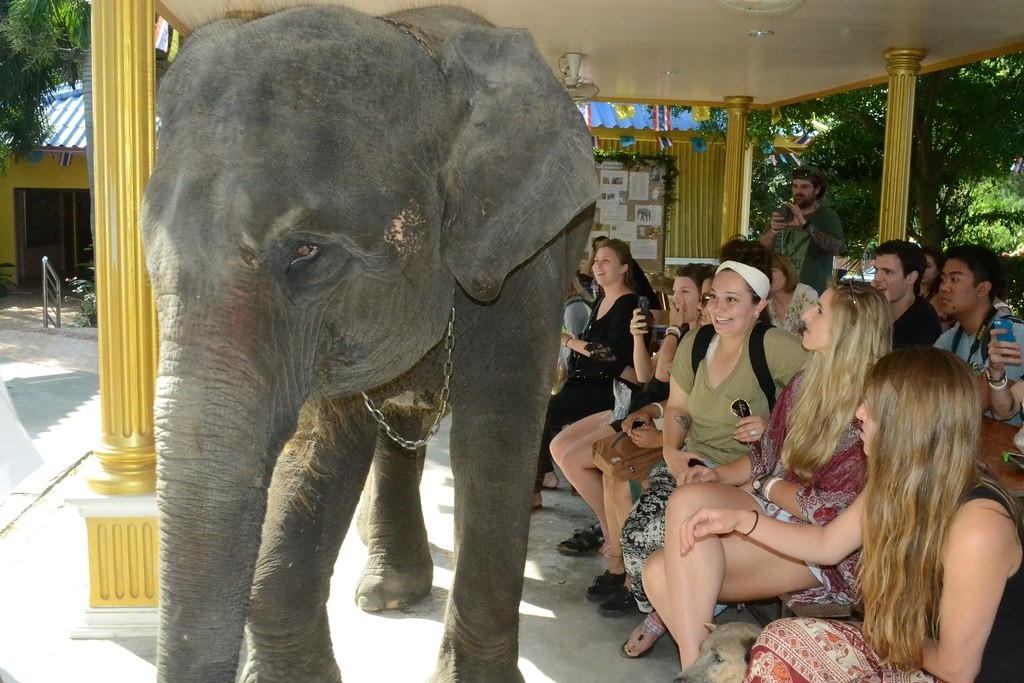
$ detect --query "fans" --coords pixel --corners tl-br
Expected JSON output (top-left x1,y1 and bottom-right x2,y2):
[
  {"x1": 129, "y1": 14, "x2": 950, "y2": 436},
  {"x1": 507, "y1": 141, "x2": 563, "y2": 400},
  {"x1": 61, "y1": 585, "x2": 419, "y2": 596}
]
[{"x1": 556, "y1": 52, "x2": 600, "y2": 100}]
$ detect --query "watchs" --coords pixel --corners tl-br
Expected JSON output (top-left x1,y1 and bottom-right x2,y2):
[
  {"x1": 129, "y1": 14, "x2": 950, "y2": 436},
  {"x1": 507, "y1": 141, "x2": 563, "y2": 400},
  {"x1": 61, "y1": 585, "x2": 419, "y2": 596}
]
[
  {"x1": 753, "y1": 473, "x2": 772, "y2": 494},
  {"x1": 985, "y1": 368, "x2": 1006, "y2": 382}
]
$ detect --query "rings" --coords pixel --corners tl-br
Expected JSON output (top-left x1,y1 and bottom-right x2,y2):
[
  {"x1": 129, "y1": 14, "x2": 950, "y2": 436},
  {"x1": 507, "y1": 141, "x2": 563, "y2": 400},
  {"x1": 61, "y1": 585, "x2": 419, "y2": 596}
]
[
  {"x1": 750, "y1": 430, "x2": 757, "y2": 436},
  {"x1": 629, "y1": 417, "x2": 633, "y2": 420},
  {"x1": 633, "y1": 323, "x2": 637, "y2": 328}
]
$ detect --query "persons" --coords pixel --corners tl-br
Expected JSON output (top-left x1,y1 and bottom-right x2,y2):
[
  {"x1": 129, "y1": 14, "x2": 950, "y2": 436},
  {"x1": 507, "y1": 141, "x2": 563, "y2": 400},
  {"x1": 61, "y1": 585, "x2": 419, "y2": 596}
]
[
  {"x1": 756, "y1": 166, "x2": 844, "y2": 297},
  {"x1": 527, "y1": 235, "x2": 1024, "y2": 683}
]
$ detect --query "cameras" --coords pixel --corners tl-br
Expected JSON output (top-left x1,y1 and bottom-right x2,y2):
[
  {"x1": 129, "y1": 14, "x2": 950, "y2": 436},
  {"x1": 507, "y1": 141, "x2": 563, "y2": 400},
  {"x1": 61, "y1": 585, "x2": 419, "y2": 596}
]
[
  {"x1": 775, "y1": 205, "x2": 791, "y2": 223},
  {"x1": 631, "y1": 421, "x2": 644, "y2": 429}
]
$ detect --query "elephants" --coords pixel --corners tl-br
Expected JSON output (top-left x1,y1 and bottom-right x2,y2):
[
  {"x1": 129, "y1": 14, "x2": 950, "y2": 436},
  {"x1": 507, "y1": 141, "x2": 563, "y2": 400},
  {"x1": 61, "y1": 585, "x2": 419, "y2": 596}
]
[{"x1": 139, "y1": 0, "x2": 599, "y2": 683}]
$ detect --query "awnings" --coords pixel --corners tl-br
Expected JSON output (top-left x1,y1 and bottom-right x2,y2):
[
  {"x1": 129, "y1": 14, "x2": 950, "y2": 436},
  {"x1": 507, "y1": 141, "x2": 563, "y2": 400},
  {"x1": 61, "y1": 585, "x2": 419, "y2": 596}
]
[{"x1": 591, "y1": 102, "x2": 819, "y2": 137}]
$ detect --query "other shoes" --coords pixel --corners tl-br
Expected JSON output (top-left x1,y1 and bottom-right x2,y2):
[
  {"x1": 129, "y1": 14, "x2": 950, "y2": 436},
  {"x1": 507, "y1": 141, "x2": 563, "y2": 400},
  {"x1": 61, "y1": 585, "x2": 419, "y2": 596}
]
[
  {"x1": 620, "y1": 615, "x2": 666, "y2": 657},
  {"x1": 586, "y1": 568, "x2": 626, "y2": 599},
  {"x1": 599, "y1": 586, "x2": 636, "y2": 616}
]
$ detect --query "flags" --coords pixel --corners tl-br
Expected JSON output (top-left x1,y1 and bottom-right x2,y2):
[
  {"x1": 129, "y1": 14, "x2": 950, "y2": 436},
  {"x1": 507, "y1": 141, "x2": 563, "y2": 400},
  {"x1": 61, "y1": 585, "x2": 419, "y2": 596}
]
[
  {"x1": 579, "y1": 103, "x2": 814, "y2": 164},
  {"x1": 154, "y1": 15, "x2": 180, "y2": 62},
  {"x1": 29, "y1": 151, "x2": 43, "y2": 162},
  {"x1": 59, "y1": 153, "x2": 72, "y2": 166}
]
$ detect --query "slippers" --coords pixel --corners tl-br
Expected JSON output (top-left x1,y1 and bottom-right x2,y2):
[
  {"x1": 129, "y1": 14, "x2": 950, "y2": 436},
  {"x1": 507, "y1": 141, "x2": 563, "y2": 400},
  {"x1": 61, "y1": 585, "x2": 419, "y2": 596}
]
[
  {"x1": 530, "y1": 503, "x2": 543, "y2": 511},
  {"x1": 557, "y1": 524, "x2": 604, "y2": 553},
  {"x1": 541, "y1": 485, "x2": 558, "y2": 491}
]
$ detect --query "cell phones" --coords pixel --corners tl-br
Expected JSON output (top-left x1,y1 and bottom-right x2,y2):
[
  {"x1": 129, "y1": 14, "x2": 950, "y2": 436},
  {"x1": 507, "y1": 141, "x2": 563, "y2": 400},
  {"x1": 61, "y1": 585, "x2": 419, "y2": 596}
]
[
  {"x1": 637, "y1": 295, "x2": 650, "y2": 331},
  {"x1": 1008, "y1": 452, "x2": 1024, "y2": 470},
  {"x1": 994, "y1": 320, "x2": 1017, "y2": 350}
]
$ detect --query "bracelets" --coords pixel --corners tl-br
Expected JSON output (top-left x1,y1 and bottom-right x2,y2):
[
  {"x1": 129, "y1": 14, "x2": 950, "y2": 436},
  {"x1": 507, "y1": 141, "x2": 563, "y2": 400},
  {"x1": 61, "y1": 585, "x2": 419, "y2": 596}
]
[
  {"x1": 666, "y1": 332, "x2": 678, "y2": 339},
  {"x1": 666, "y1": 326, "x2": 681, "y2": 338},
  {"x1": 566, "y1": 338, "x2": 572, "y2": 346},
  {"x1": 743, "y1": 509, "x2": 758, "y2": 535},
  {"x1": 988, "y1": 376, "x2": 1008, "y2": 390},
  {"x1": 652, "y1": 402, "x2": 664, "y2": 418},
  {"x1": 765, "y1": 477, "x2": 783, "y2": 501},
  {"x1": 569, "y1": 332, "x2": 575, "y2": 339},
  {"x1": 772, "y1": 229, "x2": 779, "y2": 233},
  {"x1": 802, "y1": 220, "x2": 811, "y2": 230},
  {"x1": 751, "y1": 472, "x2": 764, "y2": 486}
]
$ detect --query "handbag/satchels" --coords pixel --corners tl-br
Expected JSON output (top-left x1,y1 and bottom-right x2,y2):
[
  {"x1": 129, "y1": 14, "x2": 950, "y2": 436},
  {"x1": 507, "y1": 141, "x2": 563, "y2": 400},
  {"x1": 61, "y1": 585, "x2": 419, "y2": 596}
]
[{"x1": 594, "y1": 430, "x2": 663, "y2": 480}]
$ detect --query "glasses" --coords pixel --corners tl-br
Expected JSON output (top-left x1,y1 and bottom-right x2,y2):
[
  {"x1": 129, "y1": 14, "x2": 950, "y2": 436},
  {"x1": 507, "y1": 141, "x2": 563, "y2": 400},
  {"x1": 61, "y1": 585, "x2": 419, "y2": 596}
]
[
  {"x1": 842, "y1": 276, "x2": 856, "y2": 309},
  {"x1": 698, "y1": 296, "x2": 710, "y2": 309}
]
